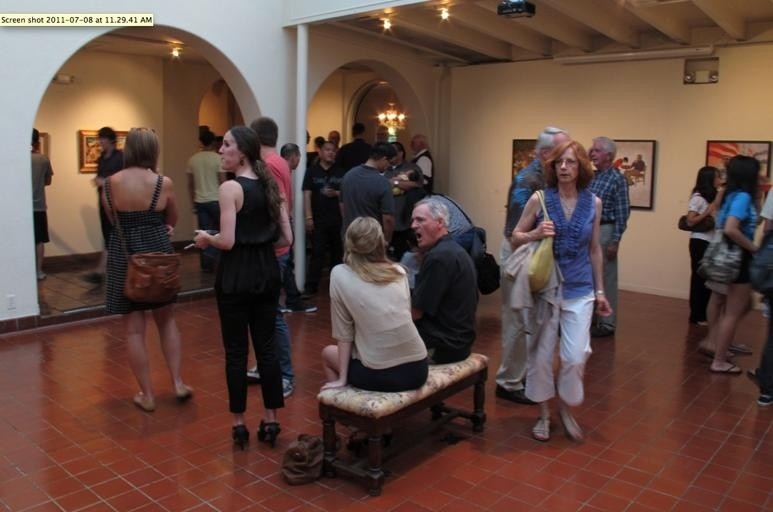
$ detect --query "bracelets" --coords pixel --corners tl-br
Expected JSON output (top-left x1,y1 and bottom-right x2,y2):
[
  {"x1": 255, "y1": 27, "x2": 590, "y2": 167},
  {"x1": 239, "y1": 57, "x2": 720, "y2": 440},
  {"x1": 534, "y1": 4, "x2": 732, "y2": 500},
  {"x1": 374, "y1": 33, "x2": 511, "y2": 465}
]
[{"x1": 595, "y1": 289, "x2": 605, "y2": 297}]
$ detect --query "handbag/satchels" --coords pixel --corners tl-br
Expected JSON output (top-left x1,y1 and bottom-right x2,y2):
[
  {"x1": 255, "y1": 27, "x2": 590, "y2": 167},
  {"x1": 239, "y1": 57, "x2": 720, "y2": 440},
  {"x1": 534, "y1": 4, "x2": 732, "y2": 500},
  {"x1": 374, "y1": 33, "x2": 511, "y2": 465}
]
[
  {"x1": 471, "y1": 227, "x2": 487, "y2": 261},
  {"x1": 678, "y1": 214, "x2": 773, "y2": 296},
  {"x1": 122, "y1": 250, "x2": 182, "y2": 303},
  {"x1": 507, "y1": 236, "x2": 554, "y2": 293},
  {"x1": 280, "y1": 433, "x2": 325, "y2": 485},
  {"x1": 475, "y1": 251, "x2": 501, "y2": 296}
]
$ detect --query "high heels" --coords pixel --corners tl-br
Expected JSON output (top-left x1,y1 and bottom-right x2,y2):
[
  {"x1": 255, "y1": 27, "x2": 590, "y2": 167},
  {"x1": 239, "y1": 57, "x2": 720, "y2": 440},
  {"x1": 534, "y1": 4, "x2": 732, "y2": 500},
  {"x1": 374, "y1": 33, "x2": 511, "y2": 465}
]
[
  {"x1": 256, "y1": 419, "x2": 282, "y2": 449},
  {"x1": 231, "y1": 424, "x2": 250, "y2": 451},
  {"x1": 346, "y1": 430, "x2": 393, "y2": 457}
]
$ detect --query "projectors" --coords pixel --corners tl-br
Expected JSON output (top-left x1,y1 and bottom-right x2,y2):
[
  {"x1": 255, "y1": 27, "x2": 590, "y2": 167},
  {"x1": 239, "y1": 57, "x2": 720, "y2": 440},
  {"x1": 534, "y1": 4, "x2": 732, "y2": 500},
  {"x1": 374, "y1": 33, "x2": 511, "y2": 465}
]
[{"x1": 498, "y1": 2, "x2": 536, "y2": 18}]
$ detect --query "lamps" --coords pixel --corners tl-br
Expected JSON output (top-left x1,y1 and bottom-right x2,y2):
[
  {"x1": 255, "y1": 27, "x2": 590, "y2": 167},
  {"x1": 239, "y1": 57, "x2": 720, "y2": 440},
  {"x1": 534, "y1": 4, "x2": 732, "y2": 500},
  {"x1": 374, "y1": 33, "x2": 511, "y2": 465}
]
[{"x1": 683, "y1": 68, "x2": 721, "y2": 84}]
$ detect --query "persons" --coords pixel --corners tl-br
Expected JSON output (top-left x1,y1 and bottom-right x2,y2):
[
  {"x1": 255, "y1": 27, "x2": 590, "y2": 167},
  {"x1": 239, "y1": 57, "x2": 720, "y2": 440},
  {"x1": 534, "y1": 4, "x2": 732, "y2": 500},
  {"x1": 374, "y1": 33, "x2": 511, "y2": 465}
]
[
  {"x1": 510, "y1": 139, "x2": 613, "y2": 443},
  {"x1": 407, "y1": 198, "x2": 479, "y2": 365},
  {"x1": 77, "y1": 127, "x2": 125, "y2": 284},
  {"x1": 586, "y1": 135, "x2": 631, "y2": 339},
  {"x1": 29, "y1": 128, "x2": 54, "y2": 281},
  {"x1": 189, "y1": 124, "x2": 293, "y2": 452},
  {"x1": 492, "y1": 126, "x2": 576, "y2": 406},
  {"x1": 99, "y1": 126, "x2": 195, "y2": 414},
  {"x1": 245, "y1": 116, "x2": 296, "y2": 399},
  {"x1": 317, "y1": 215, "x2": 430, "y2": 451},
  {"x1": 612, "y1": 156, "x2": 628, "y2": 173},
  {"x1": 686, "y1": 154, "x2": 773, "y2": 407},
  {"x1": 186, "y1": 124, "x2": 434, "y2": 314},
  {"x1": 623, "y1": 153, "x2": 646, "y2": 186}
]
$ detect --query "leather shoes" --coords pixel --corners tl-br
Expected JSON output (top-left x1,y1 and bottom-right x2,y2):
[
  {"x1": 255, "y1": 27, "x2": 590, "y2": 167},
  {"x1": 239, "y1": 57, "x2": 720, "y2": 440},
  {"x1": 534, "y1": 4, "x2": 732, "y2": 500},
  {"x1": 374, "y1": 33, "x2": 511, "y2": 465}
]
[
  {"x1": 590, "y1": 326, "x2": 615, "y2": 338},
  {"x1": 132, "y1": 391, "x2": 155, "y2": 412},
  {"x1": 176, "y1": 386, "x2": 193, "y2": 402},
  {"x1": 495, "y1": 378, "x2": 538, "y2": 405}
]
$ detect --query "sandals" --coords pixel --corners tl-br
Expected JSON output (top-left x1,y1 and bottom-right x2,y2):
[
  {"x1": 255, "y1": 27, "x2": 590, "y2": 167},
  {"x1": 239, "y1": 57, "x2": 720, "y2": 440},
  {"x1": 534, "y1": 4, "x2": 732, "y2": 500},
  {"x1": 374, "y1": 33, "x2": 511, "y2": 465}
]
[
  {"x1": 558, "y1": 410, "x2": 584, "y2": 443},
  {"x1": 531, "y1": 415, "x2": 552, "y2": 441}
]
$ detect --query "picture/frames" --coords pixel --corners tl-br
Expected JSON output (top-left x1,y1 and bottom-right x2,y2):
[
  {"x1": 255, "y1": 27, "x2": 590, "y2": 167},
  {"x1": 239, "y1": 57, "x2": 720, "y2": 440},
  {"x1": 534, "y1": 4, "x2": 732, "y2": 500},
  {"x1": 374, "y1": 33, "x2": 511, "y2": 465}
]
[
  {"x1": 703, "y1": 139, "x2": 773, "y2": 202},
  {"x1": 609, "y1": 137, "x2": 658, "y2": 211},
  {"x1": 78, "y1": 129, "x2": 129, "y2": 175},
  {"x1": 508, "y1": 137, "x2": 539, "y2": 178}
]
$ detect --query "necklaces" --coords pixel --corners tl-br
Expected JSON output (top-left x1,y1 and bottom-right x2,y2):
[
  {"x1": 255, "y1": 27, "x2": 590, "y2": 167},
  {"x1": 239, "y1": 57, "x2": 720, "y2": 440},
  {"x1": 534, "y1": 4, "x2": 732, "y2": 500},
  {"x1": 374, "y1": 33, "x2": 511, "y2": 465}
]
[{"x1": 559, "y1": 192, "x2": 576, "y2": 215}]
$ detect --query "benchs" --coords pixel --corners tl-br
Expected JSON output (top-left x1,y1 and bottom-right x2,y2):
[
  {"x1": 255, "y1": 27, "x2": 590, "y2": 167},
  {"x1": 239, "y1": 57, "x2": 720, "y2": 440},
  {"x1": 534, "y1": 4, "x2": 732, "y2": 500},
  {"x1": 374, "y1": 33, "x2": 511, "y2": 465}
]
[{"x1": 318, "y1": 353, "x2": 495, "y2": 500}]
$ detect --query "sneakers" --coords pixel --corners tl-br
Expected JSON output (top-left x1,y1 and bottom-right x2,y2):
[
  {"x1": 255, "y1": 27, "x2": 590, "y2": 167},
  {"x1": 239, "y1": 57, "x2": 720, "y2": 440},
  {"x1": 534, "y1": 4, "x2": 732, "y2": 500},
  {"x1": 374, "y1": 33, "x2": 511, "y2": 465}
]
[
  {"x1": 688, "y1": 318, "x2": 773, "y2": 407},
  {"x1": 246, "y1": 365, "x2": 261, "y2": 383},
  {"x1": 278, "y1": 290, "x2": 320, "y2": 313},
  {"x1": 282, "y1": 378, "x2": 296, "y2": 397},
  {"x1": 36, "y1": 271, "x2": 47, "y2": 281},
  {"x1": 80, "y1": 269, "x2": 107, "y2": 283}
]
